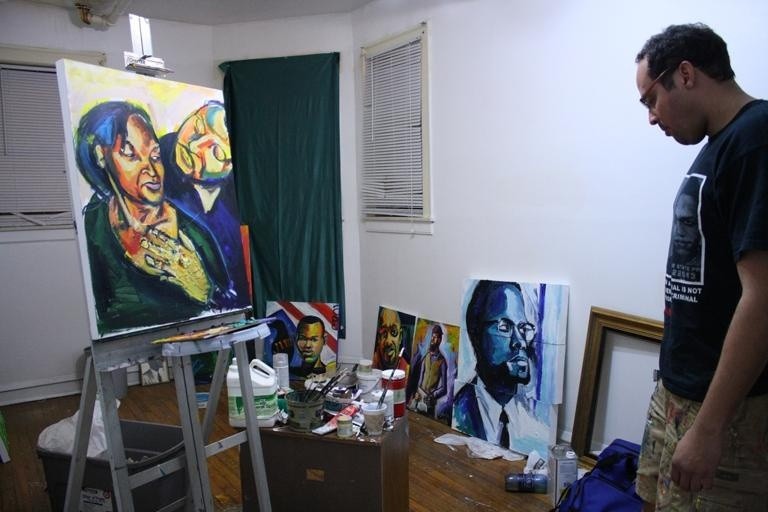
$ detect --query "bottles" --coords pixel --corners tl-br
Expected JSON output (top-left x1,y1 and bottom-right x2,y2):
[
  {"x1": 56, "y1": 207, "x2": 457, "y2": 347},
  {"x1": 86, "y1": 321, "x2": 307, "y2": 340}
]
[
  {"x1": 272, "y1": 352, "x2": 290, "y2": 389},
  {"x1": 337, "y1": 415, "x2": 353, "y2": 438},
  {"x1": 505, "y1": 473, "x2": 548, "y2": 494}
]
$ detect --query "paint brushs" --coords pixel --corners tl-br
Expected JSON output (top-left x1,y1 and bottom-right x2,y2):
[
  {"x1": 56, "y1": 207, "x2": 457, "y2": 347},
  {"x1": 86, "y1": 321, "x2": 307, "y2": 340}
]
[
  {"x1": 292, "y1": 363, "x2": 350, "y2": 403},
  {"x1": 376, "y1": 347, "x2": 405, "y2": 409}
]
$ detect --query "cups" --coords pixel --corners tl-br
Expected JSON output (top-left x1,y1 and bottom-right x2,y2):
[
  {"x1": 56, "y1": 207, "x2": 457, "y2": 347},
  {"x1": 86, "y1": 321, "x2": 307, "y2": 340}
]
[{"x1": 363, "y1": 402, "x2": 387, "y2": 435}]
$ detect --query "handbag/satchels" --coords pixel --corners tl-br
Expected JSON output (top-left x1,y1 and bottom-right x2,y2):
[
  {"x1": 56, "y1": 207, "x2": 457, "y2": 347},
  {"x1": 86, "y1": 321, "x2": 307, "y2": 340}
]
[{"x1": 556, "y1": 439, "x2": 641, "y2": 511}]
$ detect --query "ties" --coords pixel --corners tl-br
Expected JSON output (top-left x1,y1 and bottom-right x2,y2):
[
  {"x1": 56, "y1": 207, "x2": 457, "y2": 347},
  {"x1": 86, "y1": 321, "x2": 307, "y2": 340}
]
[{"x1": 499, "y1": 409, "x2": 509, "y2": 449}]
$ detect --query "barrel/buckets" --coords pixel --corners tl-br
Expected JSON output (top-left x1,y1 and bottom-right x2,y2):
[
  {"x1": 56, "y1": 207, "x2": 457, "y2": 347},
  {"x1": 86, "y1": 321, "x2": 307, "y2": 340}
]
[{"x1": 355, "y1": 367, "x2": 382, "y2": 403}]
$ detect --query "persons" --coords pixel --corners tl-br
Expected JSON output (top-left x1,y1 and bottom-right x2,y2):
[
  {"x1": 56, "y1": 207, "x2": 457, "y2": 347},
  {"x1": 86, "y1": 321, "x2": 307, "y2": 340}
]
[
  {"x1": 374, "y1": 312, "x2": 409, "y2": 386},
  {"x1": 411, "y1": 322, "x2": 448, "y2": 418},
  {"x1": 75, "y1": 100, "x2": 241, "y2": 335},
  {"x1": 274, "y1": 314, "x2": 337, "y2": 392},
  {"x1": 155, "y1": 101, "x2": 251, "y2": 308},
  {"x1": 633, "y1": 18, "x2": 766, "y2": 510},
  {"x1": 456, "y1": 281, "x2": 551, "y2": 462}
]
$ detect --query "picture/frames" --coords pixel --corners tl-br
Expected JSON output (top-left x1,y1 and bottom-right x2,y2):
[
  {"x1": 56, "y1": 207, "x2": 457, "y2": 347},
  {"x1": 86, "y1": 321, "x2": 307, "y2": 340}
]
[{"x1": 570, "y1": 305, "x2": 664, "y2": 471}]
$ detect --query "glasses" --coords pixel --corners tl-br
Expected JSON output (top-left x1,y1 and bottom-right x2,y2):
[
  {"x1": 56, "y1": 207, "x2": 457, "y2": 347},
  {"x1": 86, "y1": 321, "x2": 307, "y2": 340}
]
[
  {"x1": 640, "y1": 67, "x2": 671, "y2": 108},
  {"x1": 380, "y1": 327, "x2": 398, "y2": 338},
  {"x1": 482, "y1": 317, "x2": 536, "y2": 342}
]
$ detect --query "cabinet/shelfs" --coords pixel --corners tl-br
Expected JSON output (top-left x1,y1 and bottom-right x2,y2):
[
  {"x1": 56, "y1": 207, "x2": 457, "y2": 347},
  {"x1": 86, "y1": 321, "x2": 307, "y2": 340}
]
[{"x1": 235, "y1": 415, "x2": 410, "y2": 512}]
